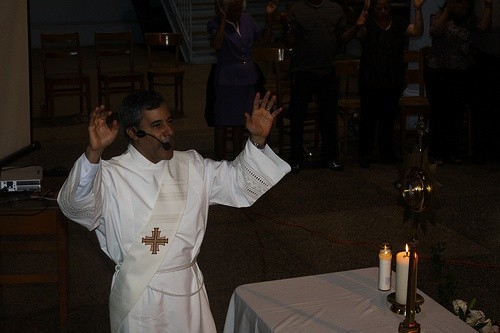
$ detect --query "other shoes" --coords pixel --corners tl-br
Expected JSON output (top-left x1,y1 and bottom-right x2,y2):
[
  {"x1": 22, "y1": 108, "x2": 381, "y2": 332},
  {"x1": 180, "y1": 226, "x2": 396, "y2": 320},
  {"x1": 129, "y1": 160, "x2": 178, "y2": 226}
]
[
  {"x1": 289, "y1": 157, "x2": 303, "y2": 171},
  {"x1": 432, "y1": 158, "x2": 445, "y2": 166},
  {"x1": 382, "y1": 154, "x2": 403, "y2": 164},
  {"x1": 325, "y1": 159, "x2": 343, "y2": 170},
  {"x1": 358, "y1": 152, "x2": 371, "y2": 168}
]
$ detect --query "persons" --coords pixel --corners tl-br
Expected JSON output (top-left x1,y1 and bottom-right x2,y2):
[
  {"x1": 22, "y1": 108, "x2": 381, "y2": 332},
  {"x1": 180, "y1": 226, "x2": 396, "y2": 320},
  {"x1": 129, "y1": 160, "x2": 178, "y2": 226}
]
[
  {"x1": 208, "y1": 0, "x2": 500, "y2": 169},
  {"x1": 57, "y1": 83, "x2": 292, "y2": 333}
]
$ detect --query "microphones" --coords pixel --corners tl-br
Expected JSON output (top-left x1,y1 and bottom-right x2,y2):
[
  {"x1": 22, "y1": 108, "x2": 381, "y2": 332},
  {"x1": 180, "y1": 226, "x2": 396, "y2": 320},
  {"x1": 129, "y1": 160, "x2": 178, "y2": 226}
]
[{"x1": 136, "y1": 130, "x2": 171, "y2": 151}]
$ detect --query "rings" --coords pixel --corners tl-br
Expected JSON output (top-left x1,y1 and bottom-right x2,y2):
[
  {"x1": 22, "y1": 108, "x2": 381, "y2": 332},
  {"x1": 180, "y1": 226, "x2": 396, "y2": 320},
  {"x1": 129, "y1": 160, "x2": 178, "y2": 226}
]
[{"x1": 266, "y1": 108, "x2": 270, "y2": 111}]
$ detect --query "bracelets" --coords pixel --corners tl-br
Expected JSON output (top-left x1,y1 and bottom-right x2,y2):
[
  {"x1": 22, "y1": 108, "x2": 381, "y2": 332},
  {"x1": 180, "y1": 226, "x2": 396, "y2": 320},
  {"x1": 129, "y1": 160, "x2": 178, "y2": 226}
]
[
  {"x1": 353, "y1": 25, "x2": 360, "y2": 32},
  {"x1": 415, "y1": 8, "x2": 422, "y2": 10},
  {"x1": 249, "y1": 136, "x2": 267, "y2": 150}
]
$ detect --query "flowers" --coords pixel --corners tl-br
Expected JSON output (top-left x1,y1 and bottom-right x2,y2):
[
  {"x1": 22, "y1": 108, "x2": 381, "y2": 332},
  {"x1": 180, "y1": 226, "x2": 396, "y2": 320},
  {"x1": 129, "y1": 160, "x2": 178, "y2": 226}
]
[{"x1": 451, "y1": 298, "x2": 500, "y2": 333}]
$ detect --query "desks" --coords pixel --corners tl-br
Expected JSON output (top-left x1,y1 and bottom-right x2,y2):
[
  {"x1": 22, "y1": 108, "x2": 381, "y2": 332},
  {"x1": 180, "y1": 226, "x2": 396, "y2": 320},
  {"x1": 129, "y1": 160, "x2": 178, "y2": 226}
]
[
  {"x1": 223, "y1": 267, "x2": 480, "y2": 333},
  {"x1": 0, "y1": 192, "x2": 70, "y2": 333}
]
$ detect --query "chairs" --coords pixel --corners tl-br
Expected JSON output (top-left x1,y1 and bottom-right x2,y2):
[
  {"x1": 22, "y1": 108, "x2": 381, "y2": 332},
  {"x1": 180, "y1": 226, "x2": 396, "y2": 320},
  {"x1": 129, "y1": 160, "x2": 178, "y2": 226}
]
[
  {"x1": 226, "y1": 48, "x2": 429, "y2": 157},
  {"x1": 41, "y1": 32, "x2": 92, "y2": 124},
  {"x1": 94, "y1": 30, "x2": 145, "y2": 112},
  {"x1": 145, "y1": 33, "x2": 185, "y2": 116}
]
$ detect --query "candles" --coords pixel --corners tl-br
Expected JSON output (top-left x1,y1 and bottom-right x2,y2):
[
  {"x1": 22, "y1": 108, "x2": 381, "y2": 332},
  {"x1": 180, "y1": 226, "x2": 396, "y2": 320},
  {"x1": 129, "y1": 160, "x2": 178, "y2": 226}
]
[
  {"x1": 379, "y1": 245, "x2": 392, "y2": 290},
  {"x1": 396, "y1": 244, "x2": 418, "y2": 305}
]
